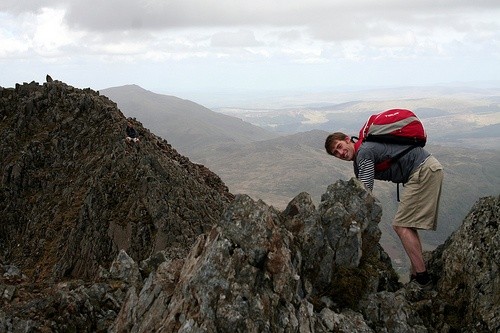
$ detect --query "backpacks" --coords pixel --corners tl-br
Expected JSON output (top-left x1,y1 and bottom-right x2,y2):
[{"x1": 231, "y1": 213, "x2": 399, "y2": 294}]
[{"x1": 350, "y1": 109, "x2": 427, "y2": 171}]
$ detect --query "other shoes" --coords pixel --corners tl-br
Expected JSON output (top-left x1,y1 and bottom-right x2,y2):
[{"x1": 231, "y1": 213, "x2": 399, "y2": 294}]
[{"x1": 407, "y1": 279, "x2": 433, "y2": 291}]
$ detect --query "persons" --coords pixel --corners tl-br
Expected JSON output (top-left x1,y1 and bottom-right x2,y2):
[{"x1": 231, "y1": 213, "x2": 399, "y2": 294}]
[{"x1": 325, "y1": 133, "x2": 442, "y2": 291}]
